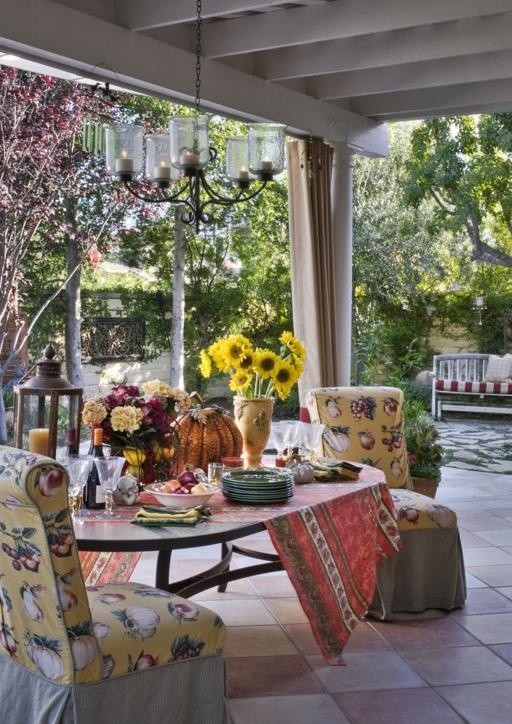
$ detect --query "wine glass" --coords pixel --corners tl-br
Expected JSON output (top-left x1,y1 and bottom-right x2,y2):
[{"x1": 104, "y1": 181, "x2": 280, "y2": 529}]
[
  {"x1": 93, "y1": 455, "x2": 127, "y2": 520},
  {"x1": 60, "y1": 454, "x2": 93, "y2": 526},
  {"x1": 271, "y1": 420, "x2": 327, "y2": 467}
]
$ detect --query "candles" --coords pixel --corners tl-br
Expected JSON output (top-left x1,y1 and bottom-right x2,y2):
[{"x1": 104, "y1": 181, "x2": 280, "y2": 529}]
[
  {"x1": 154, "y1": 161, "x2": 171, "y2": 178},
  {"x1": 115, "y1": 150, "x2": 133, "y2": 172},
  {"x1": 239, "y1": 165, "x2": 250, "y2": 178},
  {"x1": 29, "y1": 428, "x2": 50, "y2": 455},
  {"x1": 179, "y1": 150, "x2": 197, "y2": 164},
  {"x1": 259, "y1": 157, "x2": 272, "y2": 170}
]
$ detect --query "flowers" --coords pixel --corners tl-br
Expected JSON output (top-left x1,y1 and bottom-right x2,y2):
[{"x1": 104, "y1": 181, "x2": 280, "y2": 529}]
[
  {"x1": 404, "y1": 394, "x2": 446, "y2": 478},
  {"x1": 81, "y1": 364, "x2": 192, "y2": 481},
  {"x1": 199, "y1": 330, "x2": 308, "y2": 401}
]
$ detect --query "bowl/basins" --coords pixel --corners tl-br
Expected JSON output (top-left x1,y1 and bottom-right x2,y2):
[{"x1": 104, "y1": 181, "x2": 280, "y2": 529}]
[{"x1": 143, "y1": 482, "x2": 216, "y2": 509}]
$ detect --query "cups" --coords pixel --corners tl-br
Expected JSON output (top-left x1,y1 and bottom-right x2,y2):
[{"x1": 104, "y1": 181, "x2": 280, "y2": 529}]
[{"x1": 208, "y1": 462, "x2": 225, "y2": 484}]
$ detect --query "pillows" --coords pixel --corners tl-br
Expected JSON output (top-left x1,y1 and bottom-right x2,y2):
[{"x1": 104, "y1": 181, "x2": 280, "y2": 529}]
[{"x1": 485, "y1": 353, "x2": 512, "y2": 383}]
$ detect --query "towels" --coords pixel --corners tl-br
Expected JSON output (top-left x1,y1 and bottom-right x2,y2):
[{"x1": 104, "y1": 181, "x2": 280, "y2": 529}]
[
  {"x1": 130, "y1": 505, "x2": 212, "y2": 527},
  {"x1": 307, "y1": 455, "x2": 362, "y2": 483}
]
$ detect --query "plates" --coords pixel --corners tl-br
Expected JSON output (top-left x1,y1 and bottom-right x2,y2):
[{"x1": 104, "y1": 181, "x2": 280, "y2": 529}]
[{"x1": 219, "y1": 469, "x2": 296, "y2": 504}]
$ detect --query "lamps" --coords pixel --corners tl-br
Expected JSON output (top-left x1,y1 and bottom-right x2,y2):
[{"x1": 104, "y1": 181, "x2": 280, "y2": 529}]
[
  {"x1": 13, "y1": 344, "x2": 81, "y2": 460},
  {"x1": 103, "y1": 0, "x2": 287, "y2": 235},
  {"x1": 472, "y1": 292, "x2": 488, "y2": 326}
]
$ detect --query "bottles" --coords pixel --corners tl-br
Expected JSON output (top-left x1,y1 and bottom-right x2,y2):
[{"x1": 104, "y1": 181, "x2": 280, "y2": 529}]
[{"x1": 83, "y1": 427, "x2": 109, "y2": 511}]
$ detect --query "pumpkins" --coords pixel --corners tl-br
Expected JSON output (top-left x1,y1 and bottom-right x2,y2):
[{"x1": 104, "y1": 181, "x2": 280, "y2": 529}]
[{"x1": 169, "y1": 391, "x2": 242, "y2": 475}]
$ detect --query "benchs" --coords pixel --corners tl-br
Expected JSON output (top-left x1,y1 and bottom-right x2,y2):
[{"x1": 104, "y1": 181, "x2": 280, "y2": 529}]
[{"x1": 429, "y1": 353, "x2": 512, "y2": 421}]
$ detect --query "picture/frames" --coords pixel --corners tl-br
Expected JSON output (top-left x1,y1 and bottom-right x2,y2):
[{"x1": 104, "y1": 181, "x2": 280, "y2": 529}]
[{"x1": 412, "y1": 477, "x2": 442, "y2": 499}]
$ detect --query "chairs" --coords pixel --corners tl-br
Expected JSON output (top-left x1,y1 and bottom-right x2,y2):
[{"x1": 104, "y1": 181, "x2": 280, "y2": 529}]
[
  {"x1": 305, "y1": 385, "x2": 466, "y2": 618},
  {"x1": 0, "y1": 444, "x2": 230, "y2": 724}
]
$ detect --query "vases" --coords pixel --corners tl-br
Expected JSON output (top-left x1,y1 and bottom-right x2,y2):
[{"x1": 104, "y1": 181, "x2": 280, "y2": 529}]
[{"x1": 234, "y1": 395, "x2": 274, "y2": 467}]
